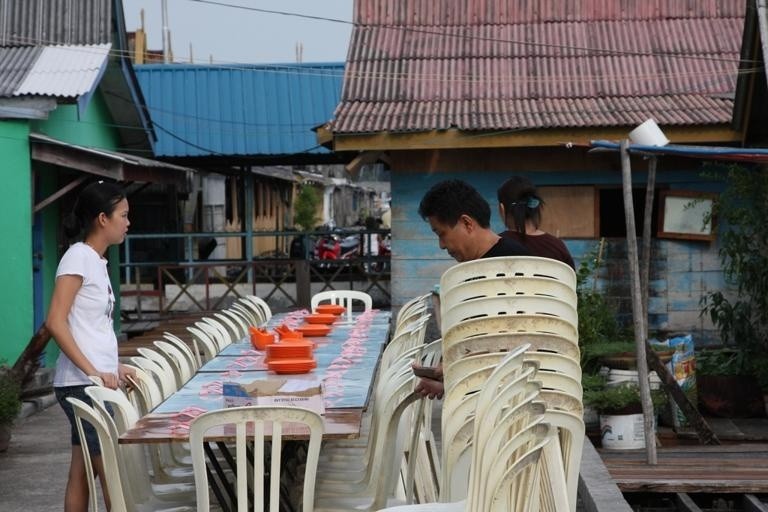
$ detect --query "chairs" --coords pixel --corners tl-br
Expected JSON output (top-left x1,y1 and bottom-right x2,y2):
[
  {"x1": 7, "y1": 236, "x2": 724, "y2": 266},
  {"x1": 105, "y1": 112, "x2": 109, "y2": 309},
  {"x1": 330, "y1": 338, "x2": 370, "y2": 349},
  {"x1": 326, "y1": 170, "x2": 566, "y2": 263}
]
[{"x1": 66, "y1": 256, "x2": 585, "y2": 512}]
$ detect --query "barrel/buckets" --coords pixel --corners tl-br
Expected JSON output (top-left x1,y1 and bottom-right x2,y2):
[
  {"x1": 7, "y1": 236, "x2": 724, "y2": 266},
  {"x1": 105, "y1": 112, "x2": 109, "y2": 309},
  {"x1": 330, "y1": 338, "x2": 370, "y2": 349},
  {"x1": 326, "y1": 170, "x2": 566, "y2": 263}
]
[
  {"x1": 582, "y1": 367, "x2": 662, "y2": 450},
  {"x1": 627, "y1": 117, "x2": 670, "y2": 147}
]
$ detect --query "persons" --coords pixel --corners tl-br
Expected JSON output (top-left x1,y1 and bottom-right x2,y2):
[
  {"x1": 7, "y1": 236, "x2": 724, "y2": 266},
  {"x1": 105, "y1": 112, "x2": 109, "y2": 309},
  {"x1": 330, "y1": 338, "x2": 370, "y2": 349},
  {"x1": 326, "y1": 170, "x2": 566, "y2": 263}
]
[
  {"x1": 407, "y1": 176, "x2": 528, "y2": 400},
  {"x1": 495, "y1": 175, "x2": 577, "y2": 274},
  {"x1": 44, "y1": 179, "x2": 130, "y2": 511}
]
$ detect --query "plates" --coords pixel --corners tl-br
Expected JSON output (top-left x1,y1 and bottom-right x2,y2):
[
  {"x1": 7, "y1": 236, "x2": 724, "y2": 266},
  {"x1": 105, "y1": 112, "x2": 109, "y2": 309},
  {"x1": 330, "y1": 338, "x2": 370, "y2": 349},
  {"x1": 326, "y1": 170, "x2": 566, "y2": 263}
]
[
  {"x1": 315, "y1": 304, "x2": 347, "y2": 314},
  {"x1": 295, "y1": 323, "x2": 331, "y2": 336},
  {"x1": 303, "y1": 313, "x2": 338, "y2": 323},
  {"x1": 263, "y1": 342, "x2": 313, "y2": 360},
  {"x1": 267, "y1": 359, "x2": 316, "y2": 374}
]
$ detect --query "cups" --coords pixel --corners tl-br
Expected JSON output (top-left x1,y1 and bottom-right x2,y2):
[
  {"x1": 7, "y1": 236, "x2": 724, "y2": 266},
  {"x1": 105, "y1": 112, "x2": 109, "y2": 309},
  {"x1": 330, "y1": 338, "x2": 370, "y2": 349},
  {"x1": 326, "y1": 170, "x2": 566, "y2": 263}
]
[
  {"x1": 278, "y1": 332, "x2": 306, "y2": 341},
  {"x1": 250, "y1": 333, "x2": 273, "y2": 349}
]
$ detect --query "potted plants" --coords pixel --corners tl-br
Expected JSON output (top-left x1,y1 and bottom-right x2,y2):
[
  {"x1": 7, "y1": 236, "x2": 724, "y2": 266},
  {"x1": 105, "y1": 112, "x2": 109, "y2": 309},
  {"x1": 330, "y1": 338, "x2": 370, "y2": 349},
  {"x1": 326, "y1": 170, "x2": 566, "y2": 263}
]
[{"x1": 588, "y1": 384, "x2": 665, "y2": 450}]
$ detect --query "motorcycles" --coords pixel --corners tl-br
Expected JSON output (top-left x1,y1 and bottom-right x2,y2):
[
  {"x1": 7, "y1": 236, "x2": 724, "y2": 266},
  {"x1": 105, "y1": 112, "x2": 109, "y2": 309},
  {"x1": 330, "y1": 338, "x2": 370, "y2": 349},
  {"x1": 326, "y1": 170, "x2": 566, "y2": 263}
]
[
  {"x1": 312, "y1": 225, "x2": 359, "y2": 261},
  {"x1": 371, "y1": 217, "x2": 392, "y2": 275}
]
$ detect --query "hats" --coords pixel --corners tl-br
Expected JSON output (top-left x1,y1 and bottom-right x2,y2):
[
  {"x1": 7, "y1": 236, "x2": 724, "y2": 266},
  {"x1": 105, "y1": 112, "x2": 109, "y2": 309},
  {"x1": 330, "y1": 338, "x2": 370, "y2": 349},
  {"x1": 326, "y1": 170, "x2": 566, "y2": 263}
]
[{"x1": 90, "y1": 179, "x2": 152, "y2": 216}]
